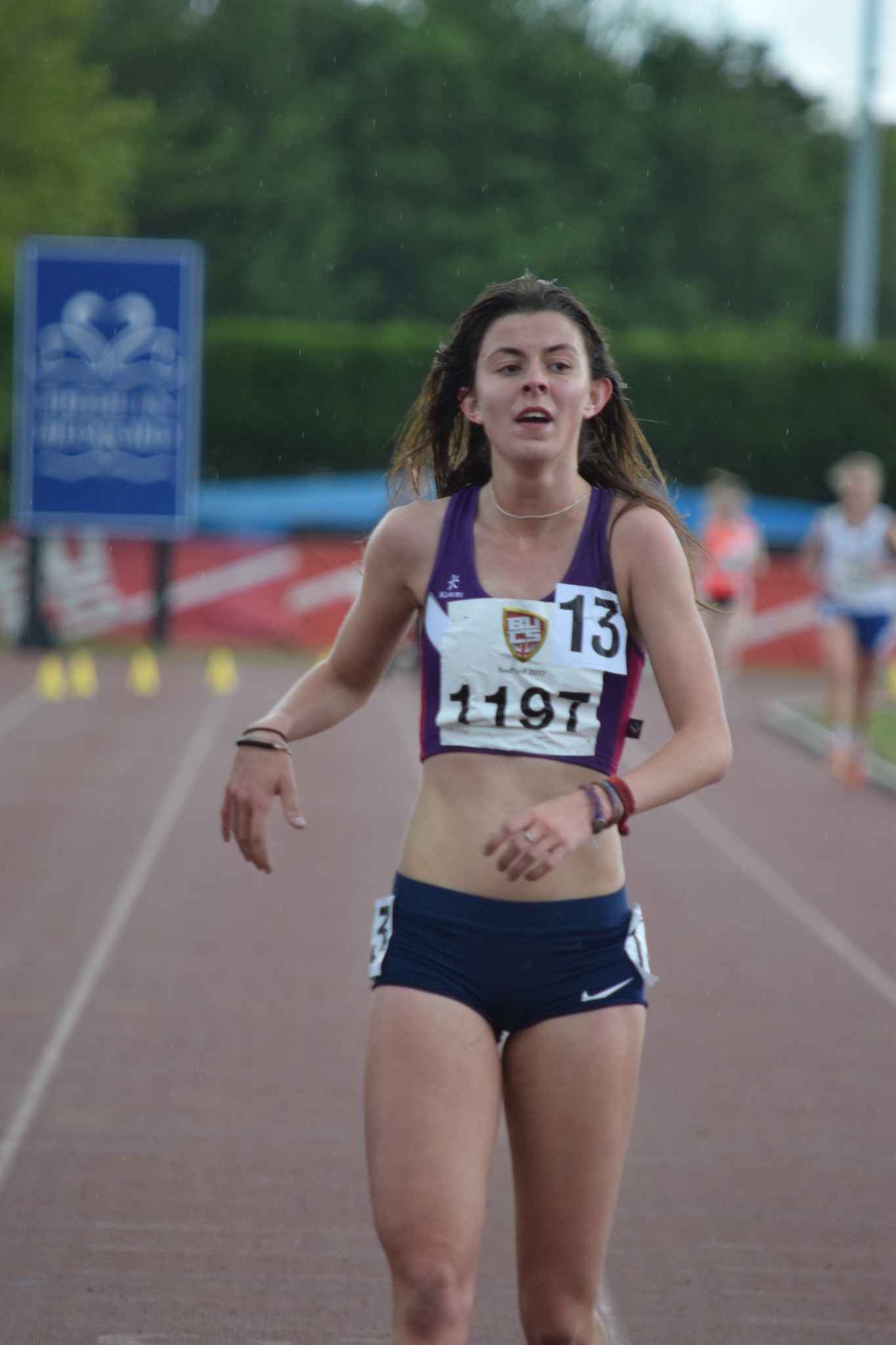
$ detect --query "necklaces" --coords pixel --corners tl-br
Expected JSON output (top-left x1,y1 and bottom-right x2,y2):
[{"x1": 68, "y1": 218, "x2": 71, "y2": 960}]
[{"x1": 489, "y1": 474, "x2": 592, "y2": 518}]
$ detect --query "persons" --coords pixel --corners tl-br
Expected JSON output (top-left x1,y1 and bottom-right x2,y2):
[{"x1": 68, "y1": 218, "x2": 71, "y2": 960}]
[
  {"x1": 689, "y1": 463, "x2": 764, "y2": 693},
  {"x1": 222, "y1": 275, "x2": 734, "y2": 1345},
  {"x1": 800, "y1": 451, "x2": 896, "y2": 790}
]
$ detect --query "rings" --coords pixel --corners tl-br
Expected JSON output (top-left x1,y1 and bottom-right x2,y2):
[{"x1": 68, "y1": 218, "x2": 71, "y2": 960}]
[{"x1": 523, "y1": 832, "x2": 535, "y2": 843}]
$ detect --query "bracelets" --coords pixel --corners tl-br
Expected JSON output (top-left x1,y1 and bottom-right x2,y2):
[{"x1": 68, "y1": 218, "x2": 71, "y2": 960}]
[
  {"x1": 604, "y1": 776, "x2": 636, "y2": 837},
  {"x1": 235, "y1": 739, "x2": 292, "y2": 754},
  {"x1": 579, "y1": 783, "x2": 608, "y2": 833},
  {"x1": 592, "y1": 779, "x2": 624, "y2": 824},
  {"x1": 242, "y1": 727, "x2": 288, "y2": 741}
]
